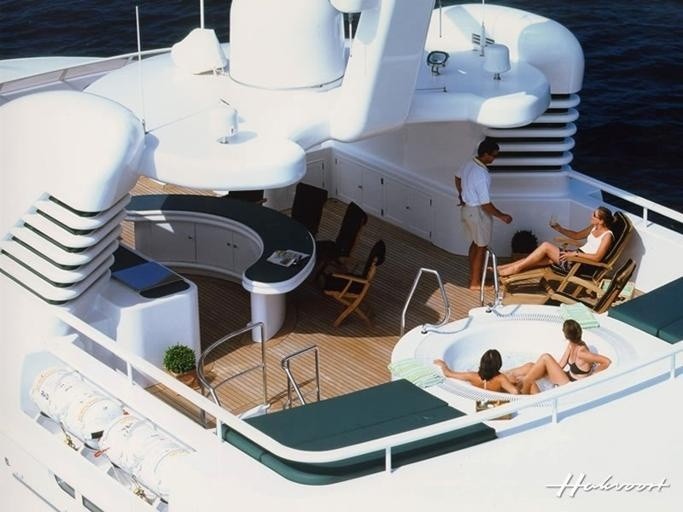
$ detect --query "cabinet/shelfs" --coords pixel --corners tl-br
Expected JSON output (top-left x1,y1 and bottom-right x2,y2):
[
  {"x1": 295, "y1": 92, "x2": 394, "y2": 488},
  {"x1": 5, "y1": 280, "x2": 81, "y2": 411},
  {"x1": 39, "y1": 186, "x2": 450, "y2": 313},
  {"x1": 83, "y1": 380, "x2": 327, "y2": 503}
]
[
  {"x1": 267, "y1": 159, "x2": 325, "y2": 211},
  {"x1": 382, "y1": 177, "x2": 431, "y2": 236},
  {"x1": 334, "y1": 154, "x2": 384, "y2": 217}
]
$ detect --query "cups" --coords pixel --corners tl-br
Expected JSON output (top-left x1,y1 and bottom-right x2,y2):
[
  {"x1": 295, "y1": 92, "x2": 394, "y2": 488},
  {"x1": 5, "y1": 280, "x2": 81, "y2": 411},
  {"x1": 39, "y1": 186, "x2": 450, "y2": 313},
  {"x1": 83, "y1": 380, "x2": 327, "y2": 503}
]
[{"x1": 549, "y1": 212, "x2": 559, "y2": 227}]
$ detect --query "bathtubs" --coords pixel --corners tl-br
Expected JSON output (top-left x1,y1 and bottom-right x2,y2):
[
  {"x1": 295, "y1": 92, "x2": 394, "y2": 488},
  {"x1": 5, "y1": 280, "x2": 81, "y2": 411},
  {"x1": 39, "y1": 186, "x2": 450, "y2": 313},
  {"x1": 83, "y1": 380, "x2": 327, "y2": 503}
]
[{"x1": 388, "y1": 304, "x2": 677, "y2": 431}]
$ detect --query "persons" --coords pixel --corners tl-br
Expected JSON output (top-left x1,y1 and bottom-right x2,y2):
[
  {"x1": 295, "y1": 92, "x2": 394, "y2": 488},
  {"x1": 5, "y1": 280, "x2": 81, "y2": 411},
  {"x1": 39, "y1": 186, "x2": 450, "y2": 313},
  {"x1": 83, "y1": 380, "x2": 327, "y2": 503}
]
[
  {"x1": 505, "y1": 320, "x2": 611, "y2": 386},
  {"x1": 487, "y1": 207, "x2": 616, "y2": 277},
  {"x1": 433, "y1": 350, "x2": 540, "y2": 395},
  {"x1": 454, "y1": 141, "x2": 513, "y2": 291}
]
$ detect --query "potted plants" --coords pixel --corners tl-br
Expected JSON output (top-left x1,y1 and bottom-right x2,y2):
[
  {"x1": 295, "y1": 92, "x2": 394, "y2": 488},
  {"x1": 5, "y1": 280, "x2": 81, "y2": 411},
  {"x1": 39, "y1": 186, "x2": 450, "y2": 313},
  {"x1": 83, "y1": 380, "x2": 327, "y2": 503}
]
[{"x1": 161, "y1": 344, "x2": 199, "y2": 389}]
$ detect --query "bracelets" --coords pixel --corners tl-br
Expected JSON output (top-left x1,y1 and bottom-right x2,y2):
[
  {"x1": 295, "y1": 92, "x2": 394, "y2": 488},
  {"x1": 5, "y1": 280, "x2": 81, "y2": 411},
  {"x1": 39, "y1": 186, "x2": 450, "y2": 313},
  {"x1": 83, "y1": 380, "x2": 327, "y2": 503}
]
[{"x1": 576, "y1": 251, "x2": 578, "y2": 257}]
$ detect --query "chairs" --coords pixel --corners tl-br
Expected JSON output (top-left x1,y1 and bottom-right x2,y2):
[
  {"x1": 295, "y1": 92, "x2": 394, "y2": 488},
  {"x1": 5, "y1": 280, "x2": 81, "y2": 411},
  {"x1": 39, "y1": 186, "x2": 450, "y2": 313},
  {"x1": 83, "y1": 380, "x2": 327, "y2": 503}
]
[
  {"x1": 310, "y1": 202, "x2": 367, "y2": 290},
  {"x1": 500, "y1": 210, "x2": 634, "y2": 288},
  {"x1": 322, "y1": 240, "x2": 387, "y2": 326},
  {"x1": 222, "y1": 190, "x2": 267, "y2": 206},
  {"x1": 286, "y1": 182, "x2": 327, "y2": 238},
  {"x1": 541, "y1": 258, "x2": 637, "y2": 314}
]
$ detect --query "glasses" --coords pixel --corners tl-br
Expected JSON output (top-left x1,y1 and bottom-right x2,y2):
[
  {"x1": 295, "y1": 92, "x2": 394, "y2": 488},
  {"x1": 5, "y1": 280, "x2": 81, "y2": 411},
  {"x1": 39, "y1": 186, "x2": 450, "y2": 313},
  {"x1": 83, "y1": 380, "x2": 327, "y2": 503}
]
[
  {"x1": 593, "y1": 212, "x2": 601, "y2": 219},
  {"x1": 488, "y1": 153, "x2": 497, "y2": 159}
]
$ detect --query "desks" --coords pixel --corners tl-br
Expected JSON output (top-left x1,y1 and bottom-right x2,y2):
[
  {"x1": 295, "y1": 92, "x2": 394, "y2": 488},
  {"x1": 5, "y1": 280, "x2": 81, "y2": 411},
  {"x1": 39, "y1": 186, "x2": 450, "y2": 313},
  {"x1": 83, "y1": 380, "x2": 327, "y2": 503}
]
[{"x1": 124, "y1": 194, "x2": 317, "y2": 342}]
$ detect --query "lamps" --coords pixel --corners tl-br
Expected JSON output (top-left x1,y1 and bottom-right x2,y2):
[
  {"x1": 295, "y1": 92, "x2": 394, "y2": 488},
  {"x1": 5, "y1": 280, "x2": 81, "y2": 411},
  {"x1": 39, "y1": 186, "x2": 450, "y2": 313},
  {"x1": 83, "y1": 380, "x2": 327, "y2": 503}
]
[{"x1": 427, "y1": 51, "x2": 449, "y2": 78}]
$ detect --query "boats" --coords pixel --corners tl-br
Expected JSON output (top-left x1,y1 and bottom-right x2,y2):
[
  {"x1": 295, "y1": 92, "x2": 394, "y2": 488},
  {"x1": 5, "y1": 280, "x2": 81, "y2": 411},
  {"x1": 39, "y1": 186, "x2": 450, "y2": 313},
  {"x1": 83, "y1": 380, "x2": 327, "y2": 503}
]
[{"x1": 0, "y1": 0, "x2": 683, "y2": 512}]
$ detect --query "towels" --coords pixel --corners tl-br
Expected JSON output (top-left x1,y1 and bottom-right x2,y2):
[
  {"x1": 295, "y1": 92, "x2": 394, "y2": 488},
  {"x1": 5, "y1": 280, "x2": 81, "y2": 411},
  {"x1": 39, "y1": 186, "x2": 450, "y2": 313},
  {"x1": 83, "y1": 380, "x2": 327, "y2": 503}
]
[
  {"x1": 560, "y1": 300, "x2": 599, "y2": 331},
  {"x1": 386, "y1": 357, "x2": 443, "y2": 389}
]
